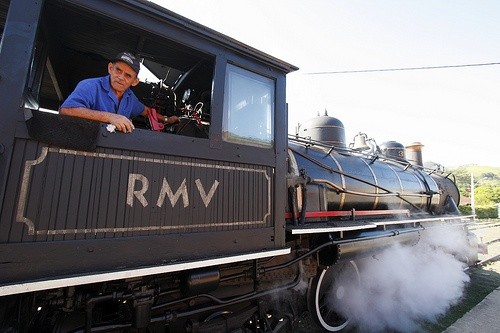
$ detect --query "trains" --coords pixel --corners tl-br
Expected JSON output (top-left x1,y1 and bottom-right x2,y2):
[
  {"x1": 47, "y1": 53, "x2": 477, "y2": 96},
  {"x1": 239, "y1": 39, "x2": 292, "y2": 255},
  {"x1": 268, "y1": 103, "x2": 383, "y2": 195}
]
[{"x1": 0, "y1": 0, "x2": 476, "y2": 333}]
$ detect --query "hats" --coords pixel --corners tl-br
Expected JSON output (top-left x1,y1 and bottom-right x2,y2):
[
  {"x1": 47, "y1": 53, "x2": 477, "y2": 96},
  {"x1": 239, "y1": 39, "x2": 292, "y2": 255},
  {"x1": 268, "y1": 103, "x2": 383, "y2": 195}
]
[{"x1": 109, "y1": 52, "x2": 140, "y2": 74}]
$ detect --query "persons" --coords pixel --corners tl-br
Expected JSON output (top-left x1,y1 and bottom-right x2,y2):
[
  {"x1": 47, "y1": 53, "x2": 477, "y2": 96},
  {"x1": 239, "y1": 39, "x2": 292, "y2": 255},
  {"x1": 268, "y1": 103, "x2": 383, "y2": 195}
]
[{"x1": 59, "y1": 51, "x2": 180, "y2": 134}]
